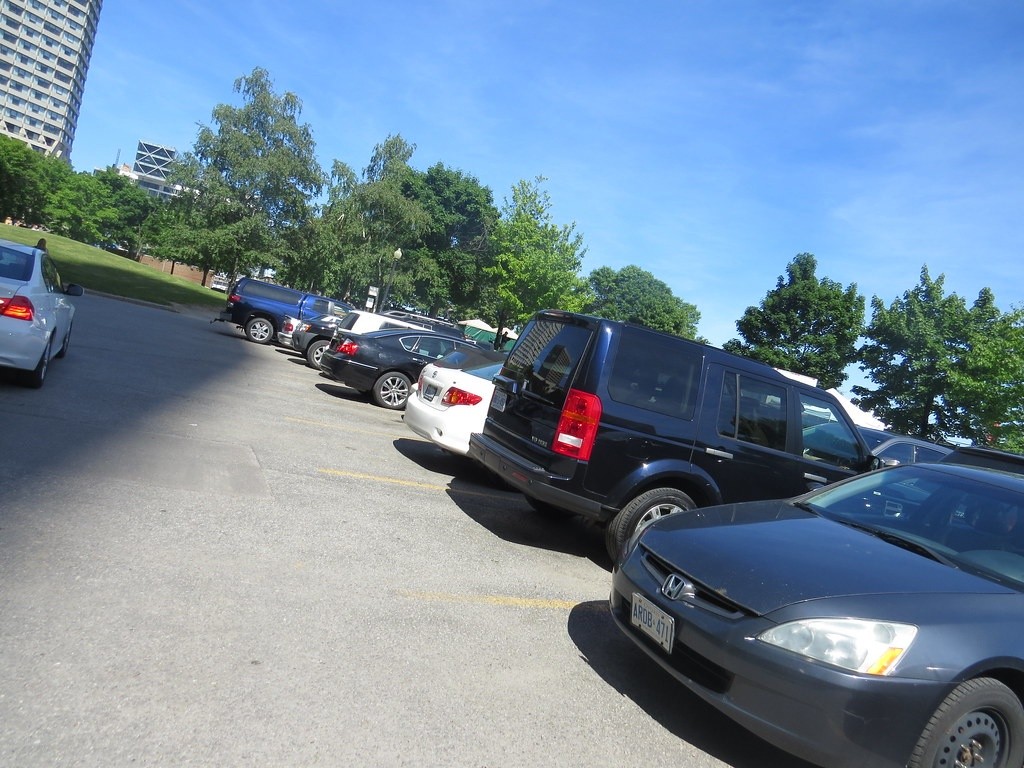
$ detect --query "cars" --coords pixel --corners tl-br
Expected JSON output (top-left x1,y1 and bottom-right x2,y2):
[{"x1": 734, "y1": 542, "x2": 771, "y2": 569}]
[
  {"x1": 609, "y1": 463, "x2": 1024, "y2": 768},
  {"x1": 0, "y1": 238, "x2": 84, "y2": 387},
  {"x1": 277, "y1": 310, "x2": 465, "y2": 370},
  {"x1": 802, "y1": 423, "x2": 954, "y2": 519},
  {"x1": 403, "y1": 348, "x2": 508, "y2": 460},
  {"x1": 319, "y1": 329, "x2": 488, "y2": 410},
  {"x1": 915, "y1": 445, "x2": 1023, "y2": 557}
]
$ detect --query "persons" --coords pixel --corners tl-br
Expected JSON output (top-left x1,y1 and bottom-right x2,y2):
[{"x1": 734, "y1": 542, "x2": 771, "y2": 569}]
[
  {"x1": 33, "y1": 238, "x2": 48, "y2": 254},
  {"x1": 5, "y1": 217, "x2": 12, "y2": 225},
  {"x1": 32, "y1": 224, "x2": 37, "y2": 230},
  {"x1": 14, "y1": 221, "x2": 23, "y2": 227}
]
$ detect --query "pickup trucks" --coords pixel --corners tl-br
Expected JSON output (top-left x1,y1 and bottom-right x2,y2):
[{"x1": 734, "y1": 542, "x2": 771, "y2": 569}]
[{"x1": 215, "y1": 278, "x2": 354, "y2": 344}]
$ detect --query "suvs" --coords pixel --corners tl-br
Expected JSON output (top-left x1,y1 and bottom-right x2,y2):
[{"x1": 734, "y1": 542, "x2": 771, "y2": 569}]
[{"x1": 469, "y1": 308, "x2": 885, "y2": 565}]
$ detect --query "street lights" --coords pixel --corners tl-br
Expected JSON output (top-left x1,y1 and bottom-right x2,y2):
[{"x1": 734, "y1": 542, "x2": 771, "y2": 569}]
[{"x1": 378, "y1": 248, "x2": 402, "y2": 312}]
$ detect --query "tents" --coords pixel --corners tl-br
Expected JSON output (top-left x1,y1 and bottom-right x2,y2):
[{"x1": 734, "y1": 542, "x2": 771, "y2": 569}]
[
  {"x1": 456, "y1": 320, "x2": 518, "y2": 352},
  {"x1": 772, "y1": 387, "x2": 884, "y2": 431}
]
